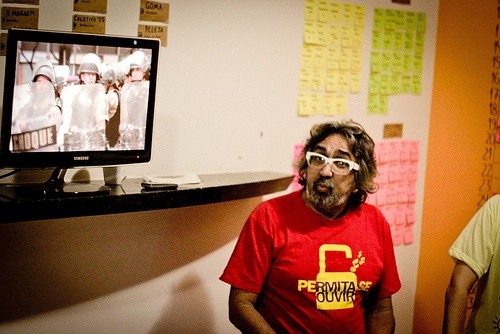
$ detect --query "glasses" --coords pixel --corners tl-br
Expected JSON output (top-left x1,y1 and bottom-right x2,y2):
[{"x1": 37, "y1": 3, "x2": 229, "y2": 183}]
[{"x1": 306, "y1": 150, "x2": 360, "y2": 176}]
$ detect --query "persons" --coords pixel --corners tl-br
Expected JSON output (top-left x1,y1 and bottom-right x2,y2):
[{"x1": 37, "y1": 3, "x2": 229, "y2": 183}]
[
  {"x1": 219, "y1": 120, "x2": 403, "y2": 334},
  {"x1": 443, "y1": 194, "x2": 500, "y2": 334},
  {"x1": 9, "y1": 51, "x2": 150, "y2": 153}
]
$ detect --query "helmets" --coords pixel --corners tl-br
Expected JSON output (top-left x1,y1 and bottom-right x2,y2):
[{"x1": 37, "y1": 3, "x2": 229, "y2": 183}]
[
  {"x1": 54, "y1": 65, "x2": 69, "y2": 86},
  {"x1": 101, "y1": 51, "x2": 151, "y2": 85},
  {"x1": 79, "y1": 62, "x2": 100, "y2": 81},
  {"x1": 33, "y1": 65, "x2": 54, "y2": 86}
]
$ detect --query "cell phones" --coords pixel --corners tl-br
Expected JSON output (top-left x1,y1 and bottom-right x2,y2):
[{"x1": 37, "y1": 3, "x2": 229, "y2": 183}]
[{"x1": 141, "y1": 182, "x2": 178, "y2": 190}]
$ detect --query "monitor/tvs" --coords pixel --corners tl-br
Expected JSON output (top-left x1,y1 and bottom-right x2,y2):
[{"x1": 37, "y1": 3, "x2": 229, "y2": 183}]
[{"x1": 1, "y1": 28, "x2": 160, "y2": 193}]
